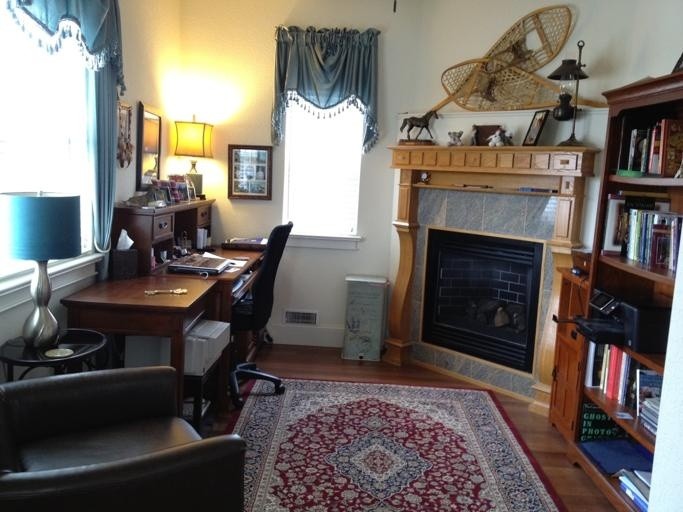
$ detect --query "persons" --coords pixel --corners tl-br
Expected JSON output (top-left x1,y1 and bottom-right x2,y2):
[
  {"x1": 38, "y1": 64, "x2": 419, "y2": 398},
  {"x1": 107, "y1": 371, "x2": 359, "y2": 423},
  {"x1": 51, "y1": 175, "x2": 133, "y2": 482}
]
[{"x1": 234, "y1": 152, "x2": 266, "y2": 194}]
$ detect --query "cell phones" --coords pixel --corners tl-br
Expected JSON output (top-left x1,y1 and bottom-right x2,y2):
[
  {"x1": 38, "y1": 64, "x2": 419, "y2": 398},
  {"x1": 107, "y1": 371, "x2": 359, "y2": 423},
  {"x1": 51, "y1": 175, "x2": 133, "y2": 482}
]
[{"x1": 233, "y1": 256, "x2": 249, "y2": 261}]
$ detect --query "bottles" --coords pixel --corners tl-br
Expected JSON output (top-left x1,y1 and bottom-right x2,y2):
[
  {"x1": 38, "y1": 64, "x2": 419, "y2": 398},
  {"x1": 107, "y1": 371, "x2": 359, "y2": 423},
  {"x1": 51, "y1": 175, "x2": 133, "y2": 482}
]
[
  {"x1": 187, "y1": 180, "x2": 195, "y2": 200},
  {"x1": 189, "y1": 160, "x2": 197, "y2": 174}
]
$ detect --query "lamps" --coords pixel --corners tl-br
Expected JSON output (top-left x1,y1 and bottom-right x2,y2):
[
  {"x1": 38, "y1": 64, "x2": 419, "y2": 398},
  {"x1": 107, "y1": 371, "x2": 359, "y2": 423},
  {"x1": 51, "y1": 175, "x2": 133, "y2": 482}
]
[
  {"x1": 547, "y1": 40, "x2": 589, "y2": 146},
  {"x1": 173, "y1": 114, "x2": 215, "y2": 200},
  {"x1": 0, "y1": 190, "x2": 81, "y2": 350}
]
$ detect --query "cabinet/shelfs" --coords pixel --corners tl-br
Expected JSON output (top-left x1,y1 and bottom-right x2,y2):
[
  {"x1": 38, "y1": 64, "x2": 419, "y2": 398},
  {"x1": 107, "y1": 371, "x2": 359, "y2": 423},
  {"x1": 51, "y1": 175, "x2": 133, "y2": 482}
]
[
  {"x1": 564, "y1": 70, "x2": 683, "y2": 512},
  {"x1": 549, "y1": 266, "x2": 589, "y2": 444}
]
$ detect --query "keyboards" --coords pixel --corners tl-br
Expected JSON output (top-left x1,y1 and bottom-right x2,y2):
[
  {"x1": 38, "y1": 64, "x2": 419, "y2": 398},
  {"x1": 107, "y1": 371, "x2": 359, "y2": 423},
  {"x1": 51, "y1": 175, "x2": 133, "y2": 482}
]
[{"x1": 231, "y1": 276, "x2": 243, "y2": 293}]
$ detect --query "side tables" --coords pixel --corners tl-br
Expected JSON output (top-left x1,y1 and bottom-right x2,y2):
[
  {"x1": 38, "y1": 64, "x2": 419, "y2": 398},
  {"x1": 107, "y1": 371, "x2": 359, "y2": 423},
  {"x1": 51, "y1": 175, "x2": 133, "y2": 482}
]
[{"x1": 0, "y1": 328, "x2": 112, "y2": 383}]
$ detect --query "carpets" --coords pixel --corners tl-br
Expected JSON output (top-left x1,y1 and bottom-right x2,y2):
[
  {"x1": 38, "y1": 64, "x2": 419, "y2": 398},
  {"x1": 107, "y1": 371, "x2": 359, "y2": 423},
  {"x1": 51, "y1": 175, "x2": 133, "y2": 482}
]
[{"x1": 225, "y1": 373, "x2": 569, "y2": 512}]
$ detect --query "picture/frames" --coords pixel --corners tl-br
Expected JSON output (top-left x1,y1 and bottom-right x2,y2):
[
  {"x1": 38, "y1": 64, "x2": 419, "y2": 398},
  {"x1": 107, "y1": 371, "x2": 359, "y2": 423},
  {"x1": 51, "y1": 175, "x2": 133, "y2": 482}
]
[
  {"x1": 522, "y1": 110, "x2": 549, "y2": 146},
  {"x1": 135, "y1": 99, "x2": 162, "y2": 193},
  {"x1": 228, "y1": 144, "x2": 273, "y2": 201},
  {"x1": 153, "y1": 181, "x2": 196, "y2": 206}
]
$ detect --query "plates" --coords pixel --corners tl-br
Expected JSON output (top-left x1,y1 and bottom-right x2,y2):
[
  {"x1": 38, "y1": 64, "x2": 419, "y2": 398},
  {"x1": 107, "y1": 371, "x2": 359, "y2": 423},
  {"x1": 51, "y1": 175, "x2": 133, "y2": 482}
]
[{"x1": 44, "y1": 348, "x2": 72, "y2": 357}]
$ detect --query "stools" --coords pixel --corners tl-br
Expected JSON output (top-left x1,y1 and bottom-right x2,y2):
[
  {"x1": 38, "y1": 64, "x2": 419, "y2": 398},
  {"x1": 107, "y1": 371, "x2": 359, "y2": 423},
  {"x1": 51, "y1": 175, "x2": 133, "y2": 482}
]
[{"x1": 180, "y1": 359, "x2": 222, "y2": 435}]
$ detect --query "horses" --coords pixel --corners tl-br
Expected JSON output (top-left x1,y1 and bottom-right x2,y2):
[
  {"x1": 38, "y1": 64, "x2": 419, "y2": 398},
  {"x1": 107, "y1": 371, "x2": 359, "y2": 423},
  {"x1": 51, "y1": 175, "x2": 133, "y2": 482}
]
[{"x1": 400, "y1": 110, "x2": 438, "y2": 140}]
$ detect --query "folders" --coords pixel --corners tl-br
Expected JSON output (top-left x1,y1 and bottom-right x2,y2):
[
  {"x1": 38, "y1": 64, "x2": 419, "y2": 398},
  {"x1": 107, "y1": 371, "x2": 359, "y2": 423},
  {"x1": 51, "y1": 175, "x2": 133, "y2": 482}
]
[{"x1": 581, "y1": 439, "x2": 651, "y2": 476}]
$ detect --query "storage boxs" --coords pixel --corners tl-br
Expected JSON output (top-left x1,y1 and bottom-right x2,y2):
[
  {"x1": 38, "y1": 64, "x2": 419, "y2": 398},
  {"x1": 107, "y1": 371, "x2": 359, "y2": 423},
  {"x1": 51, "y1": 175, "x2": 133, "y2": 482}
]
[{"x1": 122, "y1": 319, "x2": 231, "y2": 377}]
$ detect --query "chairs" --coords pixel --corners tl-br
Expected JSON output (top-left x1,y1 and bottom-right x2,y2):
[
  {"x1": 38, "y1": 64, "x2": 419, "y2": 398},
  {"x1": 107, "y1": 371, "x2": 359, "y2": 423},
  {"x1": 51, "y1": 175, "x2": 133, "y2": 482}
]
[
  {"x1": 228, "y1": 221, "x2": 294, "y2": 410},
  {"x1": 0, "y1": 364, "x2": 247, "y2": 511}
]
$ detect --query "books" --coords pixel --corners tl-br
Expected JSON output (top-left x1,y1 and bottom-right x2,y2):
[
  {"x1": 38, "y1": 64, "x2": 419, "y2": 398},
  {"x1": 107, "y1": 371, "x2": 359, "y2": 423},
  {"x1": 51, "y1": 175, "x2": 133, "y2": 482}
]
[
  {"x1": 601, "y1": 115, "x2": 683, "y2": 270},
  {"x1": 582, "y1": 341, "x2": 663, "y2": 512},
  {"x1": 161, "y1": 228, "x2": 268, "y2": 277}
]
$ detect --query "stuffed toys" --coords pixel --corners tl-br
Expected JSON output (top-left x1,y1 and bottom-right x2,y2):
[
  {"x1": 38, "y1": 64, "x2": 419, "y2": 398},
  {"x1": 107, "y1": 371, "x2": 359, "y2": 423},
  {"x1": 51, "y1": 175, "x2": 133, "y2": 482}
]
[
  {"x1": 484, "y1": 128, "x2": 512, "y2": 146},
  {"x1": 446, "y1": 129, "x2": 464, "y2": 147}
]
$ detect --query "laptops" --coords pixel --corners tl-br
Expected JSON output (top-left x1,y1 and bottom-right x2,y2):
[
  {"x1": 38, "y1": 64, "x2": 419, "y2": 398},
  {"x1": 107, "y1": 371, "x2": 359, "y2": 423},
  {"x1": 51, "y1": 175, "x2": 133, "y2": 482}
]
[{"x1": 168, "y1": 255, "x2": 230, "y2": 275}]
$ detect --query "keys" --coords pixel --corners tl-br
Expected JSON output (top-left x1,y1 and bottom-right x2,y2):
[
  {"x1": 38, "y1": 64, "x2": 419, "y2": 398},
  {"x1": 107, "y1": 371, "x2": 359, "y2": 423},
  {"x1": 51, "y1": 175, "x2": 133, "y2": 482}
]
[{"x1": 144, "y1": 288, "x2": 189, "y2": 296}]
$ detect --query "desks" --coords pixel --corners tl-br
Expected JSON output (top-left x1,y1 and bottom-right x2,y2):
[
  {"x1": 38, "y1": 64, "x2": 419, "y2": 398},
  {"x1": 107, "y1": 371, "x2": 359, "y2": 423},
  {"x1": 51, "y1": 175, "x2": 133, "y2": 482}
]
[
  {"x1": 110, "y1": 198, "x2": 266, "y2": 415},
  {"x1": 60, "y1": 275, "x2": 218, "y2": 422}
]
